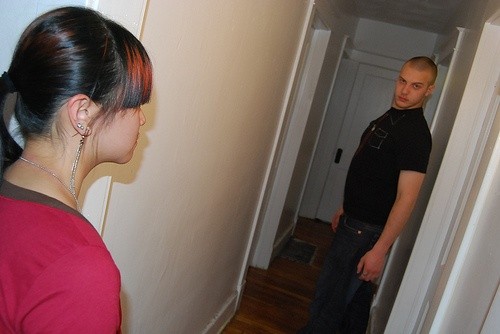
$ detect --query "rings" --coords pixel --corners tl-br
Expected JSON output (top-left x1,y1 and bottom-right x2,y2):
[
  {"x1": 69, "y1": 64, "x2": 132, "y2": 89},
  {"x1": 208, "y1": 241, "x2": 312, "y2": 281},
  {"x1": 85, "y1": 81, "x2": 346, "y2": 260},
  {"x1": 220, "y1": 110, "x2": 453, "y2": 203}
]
[{"x1": 363, "y1": 273, "x2": 366, "y2": 276}]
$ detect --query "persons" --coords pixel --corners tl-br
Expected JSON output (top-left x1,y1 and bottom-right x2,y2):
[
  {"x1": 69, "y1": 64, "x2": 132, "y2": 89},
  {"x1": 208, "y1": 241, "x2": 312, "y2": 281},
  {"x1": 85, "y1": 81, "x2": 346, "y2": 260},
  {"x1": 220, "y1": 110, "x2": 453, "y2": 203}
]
[
  {"x1": 304, "y1": 56, "x2": 438, "y2": 333},
  {"x1": 0, "y1": 5, "x2": 152, "y2": 333}
]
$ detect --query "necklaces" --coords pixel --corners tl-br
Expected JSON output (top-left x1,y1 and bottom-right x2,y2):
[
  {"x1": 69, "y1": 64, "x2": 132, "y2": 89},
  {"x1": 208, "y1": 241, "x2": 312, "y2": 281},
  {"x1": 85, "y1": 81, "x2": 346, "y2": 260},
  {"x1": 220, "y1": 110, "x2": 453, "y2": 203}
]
[
  {"x1": 18, "y1": 155, "x2": 80, "y2": 208},
  {"x1": 19, "y1": 156, "x2": 81, "y2": 212}
]
